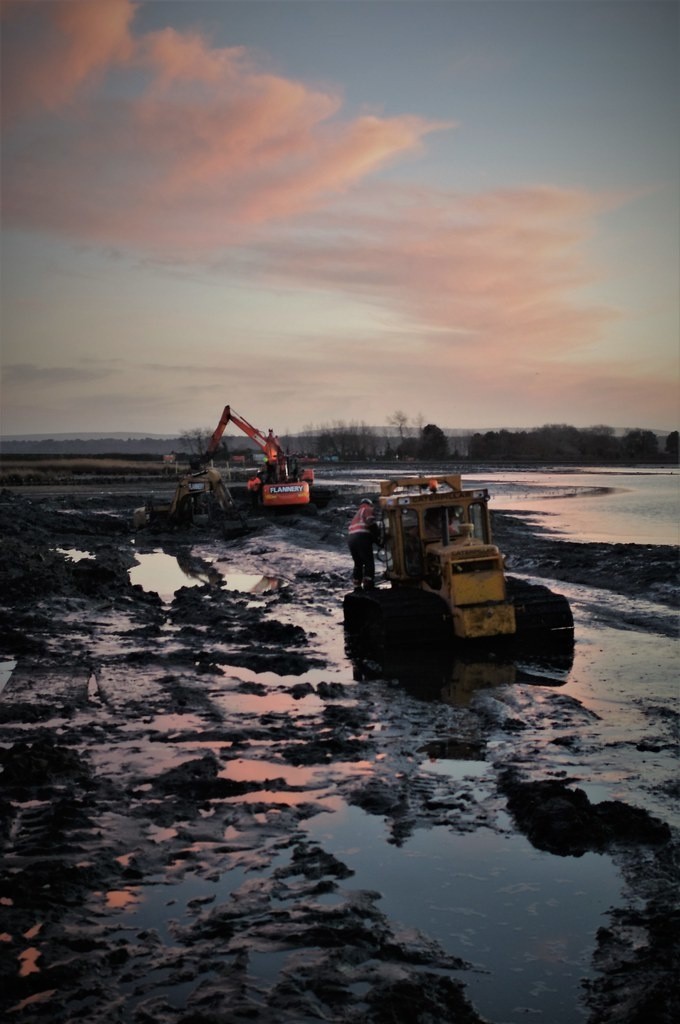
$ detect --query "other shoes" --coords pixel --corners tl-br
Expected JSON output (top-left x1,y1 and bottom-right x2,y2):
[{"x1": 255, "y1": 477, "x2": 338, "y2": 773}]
[
  {"x1": 364, "y1": 586, "x2": 380, "y2": 592},
  {"x1": 354, "y1": 587, "x2": 363, "y2": 592}
]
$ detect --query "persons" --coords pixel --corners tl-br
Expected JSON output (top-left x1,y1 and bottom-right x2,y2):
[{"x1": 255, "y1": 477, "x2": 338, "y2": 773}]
[
  {"x1": 247, "y1": 474, "x2": 262, "y2": 505},
  {"x1": 347, "y1": 498, "x2": 380, "y2": 593}
]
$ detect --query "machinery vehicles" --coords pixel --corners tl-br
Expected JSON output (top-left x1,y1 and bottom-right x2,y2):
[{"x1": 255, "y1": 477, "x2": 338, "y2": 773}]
[
  {"x1": 201, "y1": 404, "x2": 323, "y2": 518},
  {"x1": 129, "y1": 458, "x2": 251, "y2": 541},
  {"x1": 341, "y1": 471, "x2": 581, "y2": 690}
]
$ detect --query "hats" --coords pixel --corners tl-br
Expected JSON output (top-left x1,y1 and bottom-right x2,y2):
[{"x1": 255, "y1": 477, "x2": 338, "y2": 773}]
[{"x1": 360, "y1": 498, "x2": 373, "y2": 505}]
